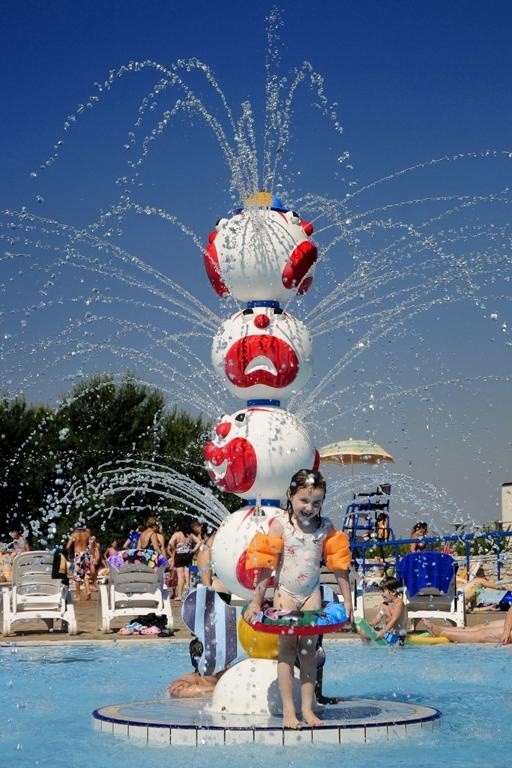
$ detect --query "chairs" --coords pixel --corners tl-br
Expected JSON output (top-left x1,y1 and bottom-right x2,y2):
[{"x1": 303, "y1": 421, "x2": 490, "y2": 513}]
[
  {"x1": 100, "y1": 550, "x2": 174, "y2": 634},
  {"x1": 320, "y1": 557, "x2": 363, "y2": 626},
  {"x1": 2, "y1": 551, "x2": 78, "y2": 636},
  {"x1": 397, "y1": 553, "x2": 465, "y2": 629}
]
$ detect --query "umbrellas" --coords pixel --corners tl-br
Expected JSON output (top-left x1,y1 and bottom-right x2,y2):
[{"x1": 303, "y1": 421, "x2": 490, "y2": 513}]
[{"x1": 316, "y1": 436, "x2": 398, "y2": 484}]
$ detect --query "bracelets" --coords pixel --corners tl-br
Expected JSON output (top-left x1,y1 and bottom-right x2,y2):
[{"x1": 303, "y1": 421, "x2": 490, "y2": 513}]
[{"x1": 479, "y1": 606, "x2": 480, "y2": 611}]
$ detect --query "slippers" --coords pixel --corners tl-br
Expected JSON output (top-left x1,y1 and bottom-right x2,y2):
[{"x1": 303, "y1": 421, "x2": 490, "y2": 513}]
[{"x1": 126, "y1": 622, "x2": 170, "y2": 637}]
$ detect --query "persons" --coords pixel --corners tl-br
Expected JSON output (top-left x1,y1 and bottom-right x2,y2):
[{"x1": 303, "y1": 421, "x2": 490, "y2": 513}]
[
  {"x1": 240, "y1": 468, "x2": 355, "y2": 728},
  {"x1": 169, "y1": 639, "x2": 225, "y2": 697},
  {"x1": 359, "y1": 576, "x2": 407, "y2": 648},
  {"x1": 463, "y1": 575, "x2": 511, "y2": 611},
  {"x1": 407, "y1": 521, "x2": 427, "y2": 552},
  {"x1": 0, "y1": 518, "x2": 222, "y2": 603},
  {"x1": 421, "y1": 605, "x2": 511, "y2": 645}
]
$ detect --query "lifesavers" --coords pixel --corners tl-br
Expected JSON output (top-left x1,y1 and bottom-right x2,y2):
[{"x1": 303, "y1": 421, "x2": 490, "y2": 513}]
[
  {"x1": 242, "y1": 598, "x2": 349, "y2": 635},
  {"x1": 405, "y1": 632, "x2": 450, "y2": 644}
]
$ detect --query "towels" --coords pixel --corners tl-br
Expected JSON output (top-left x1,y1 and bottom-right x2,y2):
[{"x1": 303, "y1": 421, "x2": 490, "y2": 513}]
[
  {"x1": 396, "y1": 552, "x2": 458, "y2": 597},
  {"x1": 51, "y1": 550, "x2": 70, "y2": 586},
  {"x1": 106, "y1": 548, "x2": 170, "y2": 573}
]
